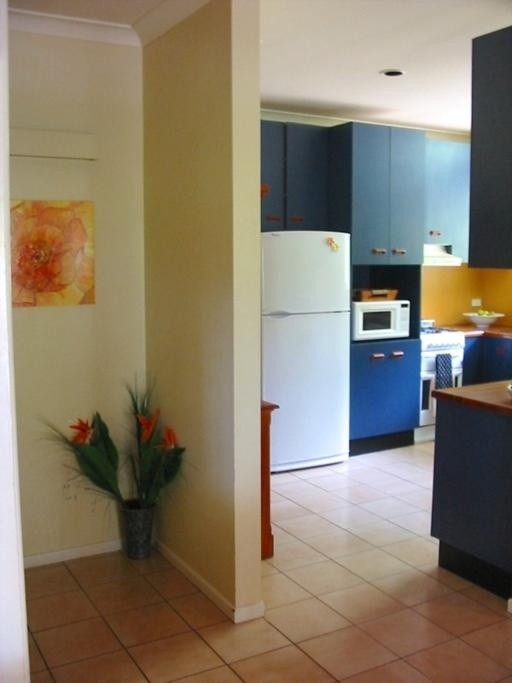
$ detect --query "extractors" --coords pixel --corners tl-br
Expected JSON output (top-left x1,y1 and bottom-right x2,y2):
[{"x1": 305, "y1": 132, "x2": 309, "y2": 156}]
[{"x1": 423, "y1": 246, "x2": 462, "y2": 267}]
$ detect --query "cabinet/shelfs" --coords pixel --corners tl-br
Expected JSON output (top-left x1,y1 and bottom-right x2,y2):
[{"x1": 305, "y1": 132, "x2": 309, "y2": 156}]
[
  {"x1": 461, "y1": 336, "x2": 512, "y2": 385},
  {"x1": 348, "y1": 338, "x2": 420, "y2": 441},
  {"x1": 260, "y1": 118, "x2": 469, "y2": 265}
]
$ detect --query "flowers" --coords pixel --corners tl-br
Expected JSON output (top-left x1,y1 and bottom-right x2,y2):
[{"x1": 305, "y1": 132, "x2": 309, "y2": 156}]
[{"x1": 44, "y1": 372, "x2": 186, "y2": 507}]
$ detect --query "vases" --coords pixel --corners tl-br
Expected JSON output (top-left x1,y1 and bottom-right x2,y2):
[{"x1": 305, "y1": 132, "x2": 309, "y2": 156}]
[{"x1": 120, "y1": 499, "x2": 157, "y2": 559}]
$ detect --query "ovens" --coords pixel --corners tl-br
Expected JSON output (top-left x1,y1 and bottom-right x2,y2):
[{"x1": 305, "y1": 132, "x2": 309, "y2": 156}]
[{"x1": 420, "y1": 337, "x2": 464, "y2": 426}]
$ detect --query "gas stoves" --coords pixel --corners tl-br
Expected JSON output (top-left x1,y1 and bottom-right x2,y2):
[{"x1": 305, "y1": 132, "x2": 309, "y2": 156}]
[{"x1": 420, "y1": 326, "x2": 463, "y2": 334}]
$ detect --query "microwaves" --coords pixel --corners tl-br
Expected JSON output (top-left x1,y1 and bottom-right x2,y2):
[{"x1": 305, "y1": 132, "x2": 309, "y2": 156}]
[{"x1": 352, "y1": 301, "x2": 409, "y2": 341}]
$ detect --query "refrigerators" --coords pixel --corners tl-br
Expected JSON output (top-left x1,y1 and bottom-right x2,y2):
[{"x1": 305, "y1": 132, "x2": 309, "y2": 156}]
[{"x1": 261, "y1": 230, "x2": 351, "y2": 475}]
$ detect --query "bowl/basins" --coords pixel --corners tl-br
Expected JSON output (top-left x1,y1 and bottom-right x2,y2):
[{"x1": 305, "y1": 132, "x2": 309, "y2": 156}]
[{"x1": 462, "y1": 312, "x2": 506, "y2": 328}]
